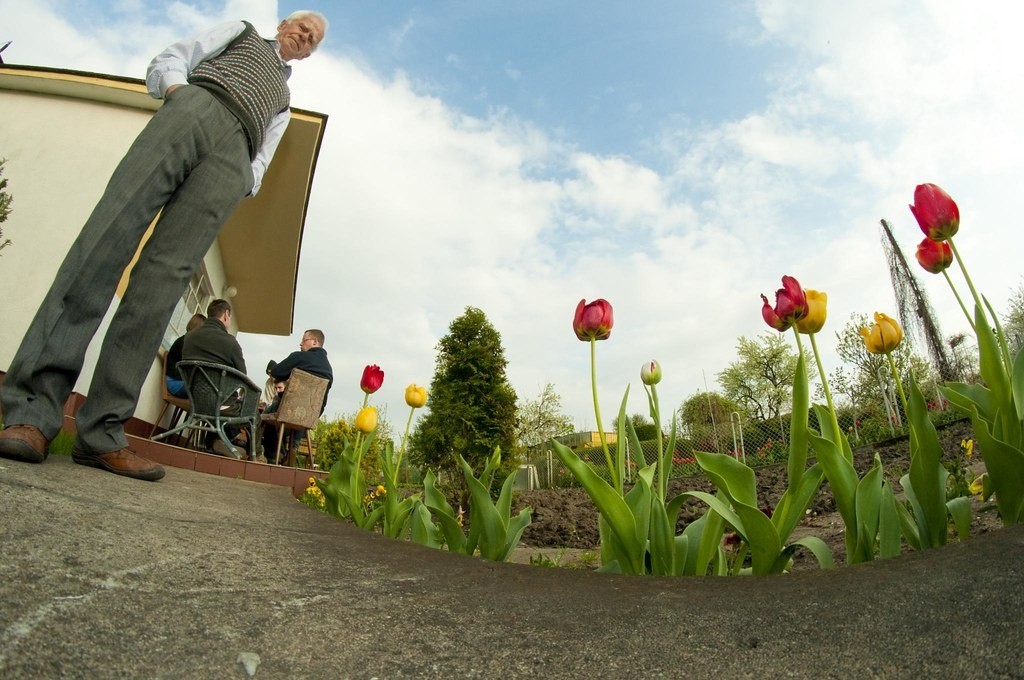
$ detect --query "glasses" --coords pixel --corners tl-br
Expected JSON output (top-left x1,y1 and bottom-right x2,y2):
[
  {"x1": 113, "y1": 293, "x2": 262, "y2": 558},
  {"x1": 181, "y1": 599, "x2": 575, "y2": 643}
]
[{"x1": 302, "y1": 338, "x2": 314, "y2": 343}]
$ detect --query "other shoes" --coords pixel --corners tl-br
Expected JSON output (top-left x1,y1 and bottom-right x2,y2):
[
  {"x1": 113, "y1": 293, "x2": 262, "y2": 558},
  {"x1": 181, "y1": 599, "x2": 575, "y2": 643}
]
[
  {"x1": 256, "y1": 452, "x2": 268, "y2": 463},
  {"x1": 212, "y1": 439, "x2": 246, "y2": 459},
  {"x1": 281, "y1": 448, "x2": 298, "y2": 466},
  {"x1": 268, "y1": 458, "x2": 276, "y2": 464}
]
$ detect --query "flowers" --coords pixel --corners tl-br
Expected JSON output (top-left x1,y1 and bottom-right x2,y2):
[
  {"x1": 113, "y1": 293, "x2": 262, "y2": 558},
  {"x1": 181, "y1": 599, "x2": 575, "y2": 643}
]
[
  {"x1": 693, "y1": 275, "x2": 836, "y2": 574},
  {"x1": 423, "y1": 445, "x2": 532, "y2": 562},
  {"x1": 340, "y1": 407, "x2": 384, "y2": 531},
  {"x1": 796, "y1": 289, "x2": 900, "y2": 564},
  {"x1": 625, "y1": 359, "x2": 771, "y2": 575},
  {"x1": 550, "y1": 299, "x2": 656, "y2": 575},
  {"x1": 295, "y1": 478, "x2": 462, "y2": 528},
  {"x1": 313, "y1": 364, "x2": 384, "y2": 521},
  {"x1": 860, "y1": 311, "x2": 972, "y2": 551},
  {"x1": 942, "y1": 439, "x2": 973, "y2": 497},
  {"x1": 379, "y1": 384, "x2": 427, "y2": 540},
  {"x1": 909, "y1": 182, "x2": 1024, "y2": 524}
]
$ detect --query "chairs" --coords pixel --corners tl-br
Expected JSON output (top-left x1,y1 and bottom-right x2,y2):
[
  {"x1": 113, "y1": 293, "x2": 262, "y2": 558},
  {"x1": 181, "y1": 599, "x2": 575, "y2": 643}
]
[{"x1": 148, "y1": 351, "x2": 330, "y2": 470}]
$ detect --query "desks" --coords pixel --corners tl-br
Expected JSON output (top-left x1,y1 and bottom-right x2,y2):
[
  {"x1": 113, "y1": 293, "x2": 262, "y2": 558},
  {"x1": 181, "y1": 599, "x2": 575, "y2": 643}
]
[{"x1": 241, "y1": 405, "x2": 267, "y2": 459}]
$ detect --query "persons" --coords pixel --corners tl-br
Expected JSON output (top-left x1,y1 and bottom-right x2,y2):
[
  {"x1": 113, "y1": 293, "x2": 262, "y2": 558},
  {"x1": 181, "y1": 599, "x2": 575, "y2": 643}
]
[
  {"x1": 0, "y1": 9, "x2": 328, "y2": 480},
  {"x1": 182, "y1": 299, "x2": 267, "y2": 464},
  {"x1": 166, "y1": 313, "x2": 333, "y2": 466}
]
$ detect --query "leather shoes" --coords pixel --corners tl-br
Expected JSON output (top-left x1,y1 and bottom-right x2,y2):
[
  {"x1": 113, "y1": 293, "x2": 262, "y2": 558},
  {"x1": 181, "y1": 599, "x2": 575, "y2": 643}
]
[
  {"x1": 71, "y1": 444, "x2": 166, "y2": 481},
  {"x1": 0, "y1": 424, "x2": 50, "y2": 463}
]
